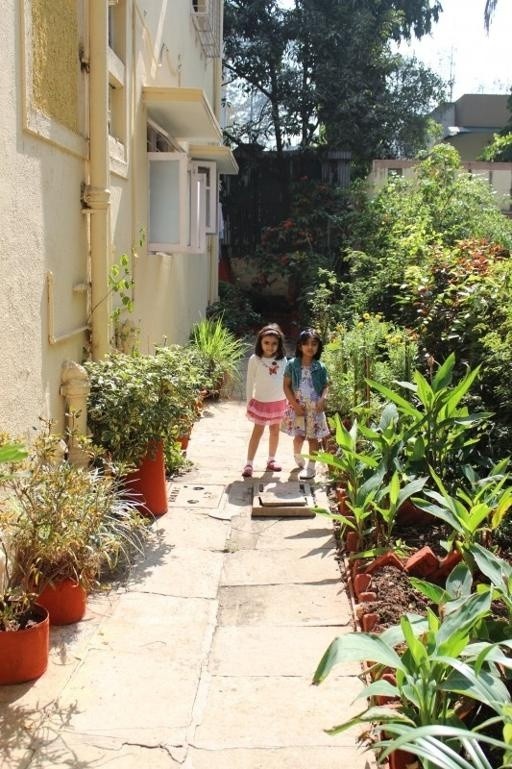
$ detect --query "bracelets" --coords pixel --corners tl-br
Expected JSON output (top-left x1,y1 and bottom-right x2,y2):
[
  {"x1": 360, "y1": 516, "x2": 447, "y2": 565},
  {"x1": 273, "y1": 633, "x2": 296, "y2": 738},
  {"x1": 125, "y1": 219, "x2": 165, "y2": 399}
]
[{"x1": 290, "y1": 399, "x2": 296, "y2": 405}]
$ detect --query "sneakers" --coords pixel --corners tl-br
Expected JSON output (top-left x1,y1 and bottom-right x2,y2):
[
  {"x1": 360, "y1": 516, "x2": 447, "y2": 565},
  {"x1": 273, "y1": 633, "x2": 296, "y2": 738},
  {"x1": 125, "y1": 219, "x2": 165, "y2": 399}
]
[
  {"x1": 242, "y1": 465, "x2": 252, "y2": 476},
  {"x1": 299, "y1": 467, "x2": 316, "y2": 478},
  {"x1": 294, "y1": 456, "x2": 305, "y2": 466},
  {"x1": 267, "y1": 460, "x2": 281, "y2": 470}
]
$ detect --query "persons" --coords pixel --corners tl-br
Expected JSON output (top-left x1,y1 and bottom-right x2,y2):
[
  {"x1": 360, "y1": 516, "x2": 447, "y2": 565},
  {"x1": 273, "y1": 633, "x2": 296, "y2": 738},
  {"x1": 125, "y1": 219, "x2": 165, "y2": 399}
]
[
  {"x1": 242, "y1": 322, "x2": 289, "y2": 477},
  {"x1": 279, "y1": 328, "x2": 332, "y2": 478}
]
